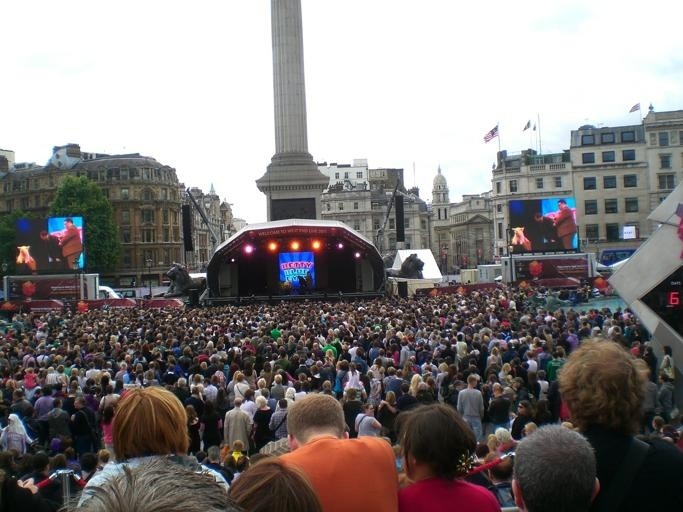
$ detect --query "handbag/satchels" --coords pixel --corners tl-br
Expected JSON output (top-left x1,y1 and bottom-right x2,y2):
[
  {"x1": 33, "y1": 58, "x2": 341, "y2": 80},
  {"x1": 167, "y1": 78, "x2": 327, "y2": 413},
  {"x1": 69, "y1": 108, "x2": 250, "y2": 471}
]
[
  {"x1": 664, "y1": 368, "x2": 675, "y2": 379},
  {"x1": 72, "y1": 429, "x2": 96, "y2": 446},
  {"x1": 264, "y1": 431, "x2": 275, "y2": 444}
]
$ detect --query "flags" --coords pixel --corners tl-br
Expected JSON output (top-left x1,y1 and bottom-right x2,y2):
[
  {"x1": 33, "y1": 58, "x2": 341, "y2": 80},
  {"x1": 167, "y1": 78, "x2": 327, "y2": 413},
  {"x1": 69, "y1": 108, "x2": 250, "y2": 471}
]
[
  {"x1": 521, "y1": 120, "x2": 528, "y2": 132},
  {"x1": 532, "y1": 122, "x2": 535, "y2": 131},
  {"x1": 483, "y1": 125, "x2": 497, "y2": 143},
  {"x1": 628, "y1": 102, "x2": 639, "y2": 112}
]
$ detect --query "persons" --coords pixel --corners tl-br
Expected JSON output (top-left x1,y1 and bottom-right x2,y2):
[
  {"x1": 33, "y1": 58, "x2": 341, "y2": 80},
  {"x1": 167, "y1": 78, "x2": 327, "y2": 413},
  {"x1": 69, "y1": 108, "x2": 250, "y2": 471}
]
[
  {"x1": 552, "y1": 199, "x2": 576, "y2": 249},
  {"x1": 533, "y1": 213, "x2": 563, "y2": 250},
  {"x1": 58, "y1": 218, "x2": 82, "y2": 270},
  {"x1": 39, "y1": 230, "x2": 63, "y2": 272},
  {"x1": 0, "y1": 274, "x2": 682, "y2": 512}
]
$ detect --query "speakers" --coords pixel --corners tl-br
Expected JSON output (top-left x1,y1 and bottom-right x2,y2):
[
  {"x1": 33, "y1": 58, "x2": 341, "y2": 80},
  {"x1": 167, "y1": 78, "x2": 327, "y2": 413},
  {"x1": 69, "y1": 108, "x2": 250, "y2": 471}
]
[
  {"x1": 182, "y1": 205, "x2": 195, "y2": 252},
  {"x1": 397, "y1": 282, "x2": 407, "y2": 297},
  {"x1": 395, "y1": 195, "x2": 404, "y2": 242},
  {"x1": 188, "y1": 290, "x2": 199, "y2": 306}
]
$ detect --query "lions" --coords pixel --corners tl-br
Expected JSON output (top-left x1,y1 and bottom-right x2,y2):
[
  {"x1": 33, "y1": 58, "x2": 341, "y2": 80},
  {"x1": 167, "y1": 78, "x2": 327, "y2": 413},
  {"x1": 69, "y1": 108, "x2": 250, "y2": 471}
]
[
  {"x1": 385, "y1": 253, "x2": 425, "y2": 280},
  {"x1": 152, "y1": 261, "x2": 207, "y2": 299}
]
